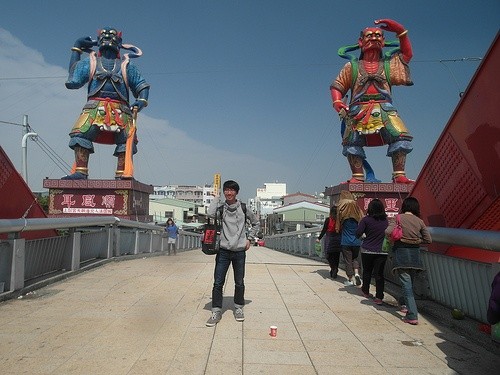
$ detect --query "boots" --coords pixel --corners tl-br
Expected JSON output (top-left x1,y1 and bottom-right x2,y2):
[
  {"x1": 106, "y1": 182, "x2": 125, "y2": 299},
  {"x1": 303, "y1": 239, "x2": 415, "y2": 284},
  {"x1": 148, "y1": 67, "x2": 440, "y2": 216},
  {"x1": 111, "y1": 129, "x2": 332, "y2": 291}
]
[{"x1": 167, "y1": 243, "x2": 176, "y2": 256}]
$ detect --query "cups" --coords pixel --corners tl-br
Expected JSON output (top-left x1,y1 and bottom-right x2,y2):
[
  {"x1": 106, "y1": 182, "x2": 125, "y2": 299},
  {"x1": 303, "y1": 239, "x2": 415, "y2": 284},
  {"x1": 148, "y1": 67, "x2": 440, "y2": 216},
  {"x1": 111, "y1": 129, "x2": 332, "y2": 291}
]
[{"x1": 270, "y1": 326, "x2": 277, "y2": 336}]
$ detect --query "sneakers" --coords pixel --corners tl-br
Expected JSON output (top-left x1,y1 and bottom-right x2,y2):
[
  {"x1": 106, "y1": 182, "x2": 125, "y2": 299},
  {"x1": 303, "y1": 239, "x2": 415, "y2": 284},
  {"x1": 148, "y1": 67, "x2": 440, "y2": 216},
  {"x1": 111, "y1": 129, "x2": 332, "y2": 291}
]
[
  {"x1": 233, "y1": 308, "x2": 244, "y2": 321},
  {"x1": 355, "y1": 274, "x2": 362, "y2": 286},
  {"x1": 360, "y1": 288, "x2": 369, "y2": 298},
  {"x1": 206, "y1": 312, "x2": 222, "y2": 326},
  {"x1": 374, "y1": 297, "x2": 383, "y2": 305},
  {"x1": 401, "y1": 316, "x2": 419, "y2": 325},
  {"x1": 397, "y1": 303, "x2": 408, "y2": 313},
  {"x1": 344, "y1": 281, "x2": 356, "y2": 286}
]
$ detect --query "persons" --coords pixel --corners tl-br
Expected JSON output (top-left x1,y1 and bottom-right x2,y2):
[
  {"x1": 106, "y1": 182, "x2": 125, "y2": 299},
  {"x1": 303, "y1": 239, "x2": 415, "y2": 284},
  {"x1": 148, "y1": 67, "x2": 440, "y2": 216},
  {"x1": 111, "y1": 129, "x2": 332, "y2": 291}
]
[
  {"x1": 488, "y1": 271, "x2": 500, "y2": 326},
  {"x1": 253, "y1": 233, "x2": 263, "y2": 246},
  {"x1": 316, "y1": 206, "x2": 343, "y2": 279},
  {"x1": 201, "y1": 180, "x2": 259, "y2": 326},
  {"x1": 336, "y1": 189, "x2": 363, "y2": 285},
  {"x1": 355, "y1": 198, "x2": 390, "y2": 305},
  {"x1": 329, "y1": 19, "x2": 414, "y2": 183},
  {"x1": 385, "y1": 196, "x2": 432, "y2": 325},
  {"x1": 60, "y1": 25, "x2": 150, "y2": 180},
  {"x1": 164, "y1": 218, "x2": 178, "y2": 256}
]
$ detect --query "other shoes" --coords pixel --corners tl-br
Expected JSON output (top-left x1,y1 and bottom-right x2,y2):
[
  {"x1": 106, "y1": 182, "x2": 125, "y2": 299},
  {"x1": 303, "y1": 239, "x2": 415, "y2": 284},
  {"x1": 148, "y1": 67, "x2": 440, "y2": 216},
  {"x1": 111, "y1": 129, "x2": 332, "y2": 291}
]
[{"x1": 329, "y1": 270, "x2": 338, "y2": 278}]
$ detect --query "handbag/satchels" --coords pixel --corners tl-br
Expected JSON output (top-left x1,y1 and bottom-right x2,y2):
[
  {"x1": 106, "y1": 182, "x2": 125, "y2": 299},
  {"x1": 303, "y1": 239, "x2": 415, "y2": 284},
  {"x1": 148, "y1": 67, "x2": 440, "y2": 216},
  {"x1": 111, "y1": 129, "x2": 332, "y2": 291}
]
[
  {"x1": 162, "y1": 228, "x2": 169, "y2": 238},
  {"x1": 387, "y1": 214, "x2": 402, "y2": 242},
  {"x1": 202, "y1": 211, "x2": 220, "y2": 255},
  {"x1": 382, "y1": 239, "x2": 391, "y2": 252}
]
